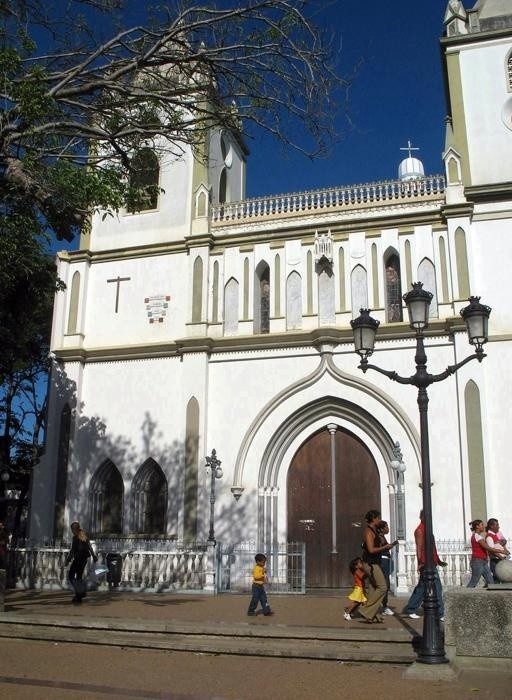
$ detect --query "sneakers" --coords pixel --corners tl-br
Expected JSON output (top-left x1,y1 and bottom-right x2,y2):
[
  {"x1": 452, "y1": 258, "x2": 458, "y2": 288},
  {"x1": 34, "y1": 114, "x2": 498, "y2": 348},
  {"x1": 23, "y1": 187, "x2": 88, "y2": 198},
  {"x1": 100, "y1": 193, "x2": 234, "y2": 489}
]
[
  {"x1": 247, "y1": 612, "x2": 258, "y2": 617},
  {"x1": 382, "y1": 608, "x2": 396, "y2": 616},
  {"x1": 70, "y1": 592, "x2": 88, "y2": 604},
  {"x1": 264, "y1": 611, "x2": 274, "y2": 617},
  {"x1": 344, "y1": 611, "x2": 352, "y2": 620},
  {"x1": 407, "y1": 613, "x2": 420, "y2": 619}
]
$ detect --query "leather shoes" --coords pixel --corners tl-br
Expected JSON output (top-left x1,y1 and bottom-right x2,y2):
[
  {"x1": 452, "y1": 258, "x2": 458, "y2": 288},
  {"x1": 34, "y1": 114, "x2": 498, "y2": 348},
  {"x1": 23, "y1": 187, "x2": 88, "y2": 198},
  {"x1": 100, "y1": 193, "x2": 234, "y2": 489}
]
[{"x1": 360, "y1": 614, "x2": 383, "y2": 624}]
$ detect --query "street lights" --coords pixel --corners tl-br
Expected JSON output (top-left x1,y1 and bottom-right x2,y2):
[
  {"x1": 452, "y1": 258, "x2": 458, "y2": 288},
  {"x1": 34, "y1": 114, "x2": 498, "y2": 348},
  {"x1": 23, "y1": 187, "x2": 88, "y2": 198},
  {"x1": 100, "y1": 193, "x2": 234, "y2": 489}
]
[
  {"x1": 349, "y1": 279, "x2": 490, "y2": 680},
  {"x1": 205, "y1": 446, "x2": 224, "y2": 595}
]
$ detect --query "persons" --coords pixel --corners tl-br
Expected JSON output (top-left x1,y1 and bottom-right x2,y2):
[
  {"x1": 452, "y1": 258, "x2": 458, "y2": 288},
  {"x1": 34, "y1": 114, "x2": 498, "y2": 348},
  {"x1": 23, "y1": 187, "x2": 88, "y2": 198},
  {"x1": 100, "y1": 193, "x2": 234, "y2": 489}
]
[
  {"x1": 61, "y1": 522, "x2": 101, "y2": 603},
  {"x1": 343, "y1": 510, "x2": 395, "y2": 624},
  {"x1": 82, "y1": 532, "x2": 99, "y2": 592},
  {"x1": 400, "y1": 510, "x2": 448, "y2": 622},
  {"x1": 0, "y1": 505, "x2": 28, "y2": 589},
  {"x1": 467, "y1": 517, "x2": 509, "y2": 587},
  {"x1": 247, "y1": 554, "x2": 274, "y2": 616}
]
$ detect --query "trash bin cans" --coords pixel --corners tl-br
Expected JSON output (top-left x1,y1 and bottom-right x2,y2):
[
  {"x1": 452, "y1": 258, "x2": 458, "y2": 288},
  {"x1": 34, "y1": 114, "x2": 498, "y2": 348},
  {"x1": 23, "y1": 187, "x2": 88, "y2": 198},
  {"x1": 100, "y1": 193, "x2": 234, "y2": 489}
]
[{"x1": 105, "y1": 552, "x2": 125, "y2": 587}]
